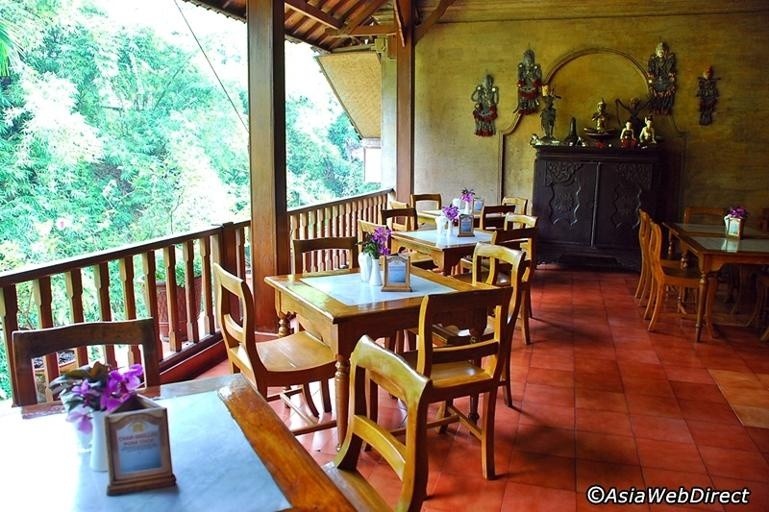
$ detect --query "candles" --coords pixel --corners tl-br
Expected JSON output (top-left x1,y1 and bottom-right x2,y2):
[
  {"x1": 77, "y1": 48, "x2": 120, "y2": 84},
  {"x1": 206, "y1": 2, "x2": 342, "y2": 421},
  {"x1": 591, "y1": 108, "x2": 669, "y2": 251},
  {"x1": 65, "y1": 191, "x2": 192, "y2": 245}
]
[{"x1": 542, "y1": 84, "x2": 549, "y2": 96}]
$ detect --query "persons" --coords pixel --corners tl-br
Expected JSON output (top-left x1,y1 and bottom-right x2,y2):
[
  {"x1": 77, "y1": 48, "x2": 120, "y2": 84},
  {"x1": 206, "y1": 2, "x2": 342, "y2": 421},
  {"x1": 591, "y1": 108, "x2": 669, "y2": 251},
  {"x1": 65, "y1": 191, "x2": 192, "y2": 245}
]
[
  {"x1": 584, "y1": 98, "x2": 615, "y2": 134},
  {"x1": 470, "y1": 75, "x2": 499, "y2": 137},
  {"x1": 696, "y1": 67, "x2": 720, "y2": 126},
  {"x1": 638, "y1": 119, "x2": 657, "y2": 150},
  {"x1": 618, "y1": 121, "x2": 637, "y2": 150},
  {"x1": 616, "y1": 98, "x2": 650, "y2": 139},
  {"x1": 539, "y1": 100, "x2": 556, "y2": 139},
  {"x1": 646, "y1": 42, "x2": 679, "y2": 115},
  {"x1": 517, "y1": 50, "x2": 542, "y2": 115}
]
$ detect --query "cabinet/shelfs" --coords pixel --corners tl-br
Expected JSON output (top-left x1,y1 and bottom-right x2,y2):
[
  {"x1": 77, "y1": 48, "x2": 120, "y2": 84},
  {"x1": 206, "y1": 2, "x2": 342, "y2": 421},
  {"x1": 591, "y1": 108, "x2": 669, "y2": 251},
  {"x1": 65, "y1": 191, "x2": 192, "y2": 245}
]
[{"x1": 528, "y1": 141, "x2": 669, "y2": 276}]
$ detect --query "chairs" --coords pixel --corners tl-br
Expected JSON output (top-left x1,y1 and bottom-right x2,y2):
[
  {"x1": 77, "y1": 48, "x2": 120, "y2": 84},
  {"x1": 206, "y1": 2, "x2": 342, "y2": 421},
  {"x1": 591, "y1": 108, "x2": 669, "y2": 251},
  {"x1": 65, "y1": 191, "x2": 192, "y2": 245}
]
[
  {"x1": 0, "y1": 191, "x2": 540, "y2": 511},
  {"x1": 630, "y1": 200, "x2": 768, "y2": 343}
]
